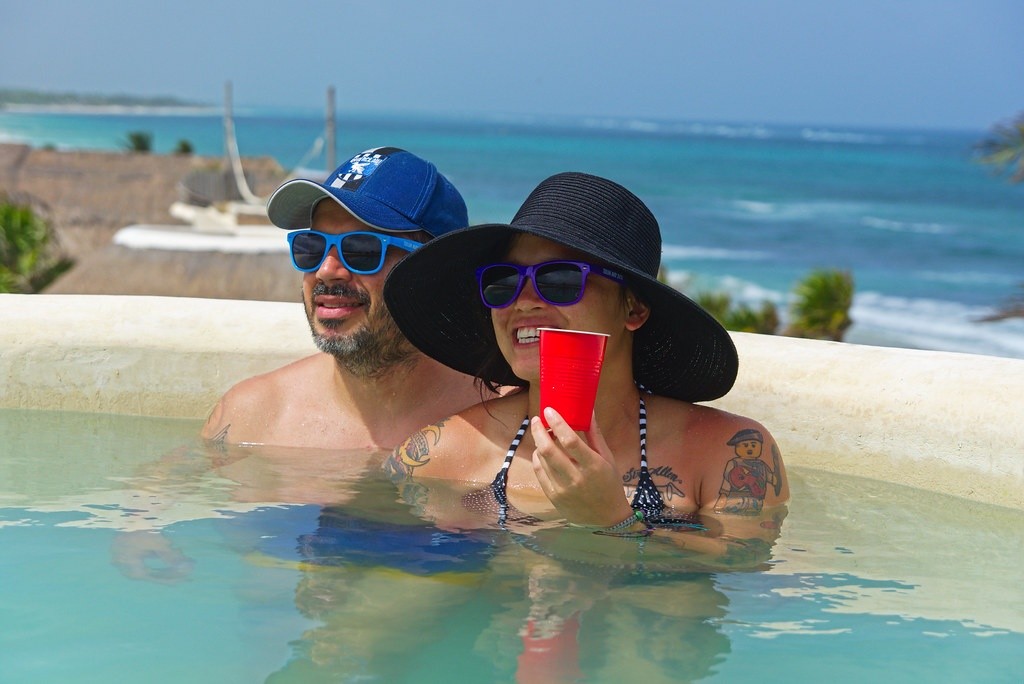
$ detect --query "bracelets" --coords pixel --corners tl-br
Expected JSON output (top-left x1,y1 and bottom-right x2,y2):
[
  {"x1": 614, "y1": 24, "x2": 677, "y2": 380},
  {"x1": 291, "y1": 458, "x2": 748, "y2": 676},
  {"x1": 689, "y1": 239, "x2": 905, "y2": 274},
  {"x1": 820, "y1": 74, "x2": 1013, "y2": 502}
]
[{"x1": 605, "y1": 511, "x2": 644, "y2": 531}]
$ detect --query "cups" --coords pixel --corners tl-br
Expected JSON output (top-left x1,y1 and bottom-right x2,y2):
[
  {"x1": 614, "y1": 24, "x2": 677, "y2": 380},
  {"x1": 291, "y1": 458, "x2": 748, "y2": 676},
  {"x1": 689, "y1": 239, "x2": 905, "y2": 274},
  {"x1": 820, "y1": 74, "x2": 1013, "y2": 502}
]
[{"x1": 536, "y1": 328, "x2": 610, "y2": 431}]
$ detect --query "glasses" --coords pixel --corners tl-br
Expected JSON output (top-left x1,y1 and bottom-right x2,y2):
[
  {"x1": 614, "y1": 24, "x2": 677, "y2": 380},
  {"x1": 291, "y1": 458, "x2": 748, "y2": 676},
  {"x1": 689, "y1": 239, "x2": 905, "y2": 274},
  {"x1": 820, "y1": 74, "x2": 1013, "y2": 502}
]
[
  {"x1": 287, "y1": 230, "x2": 425, "y2": 275},
  {"x1": 473, "y1": 259, "x2": 640, "y2": 309}
]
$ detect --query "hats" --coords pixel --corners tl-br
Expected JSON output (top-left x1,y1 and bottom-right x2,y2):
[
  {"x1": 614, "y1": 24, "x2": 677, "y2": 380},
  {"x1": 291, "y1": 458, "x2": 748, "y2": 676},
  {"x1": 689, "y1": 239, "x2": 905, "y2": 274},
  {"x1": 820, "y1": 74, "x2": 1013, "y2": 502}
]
[
  {"x1": 382, "y1": 172, "x2": 740, "y2": 404},
  {"x1": 267, "y1": 146, "x2": 469, "y2": 237}
]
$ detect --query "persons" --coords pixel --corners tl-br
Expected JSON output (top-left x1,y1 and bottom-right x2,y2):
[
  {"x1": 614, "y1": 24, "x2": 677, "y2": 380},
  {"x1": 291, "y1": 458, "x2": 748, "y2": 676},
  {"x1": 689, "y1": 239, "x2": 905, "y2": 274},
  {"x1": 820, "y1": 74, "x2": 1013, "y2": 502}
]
[
  {"x1": 105, "y1": 144, "x2": 522, "y2": 609},
  {"x1": 261, "y1": 169, "x2": 787, "y2": 679}
]
